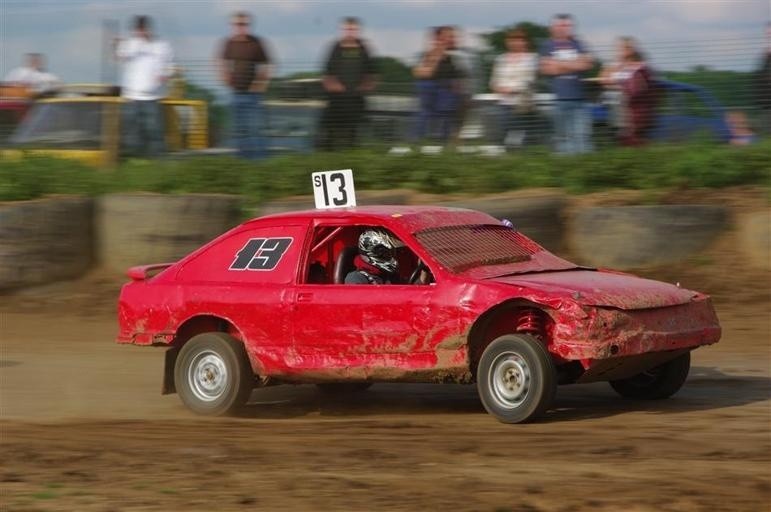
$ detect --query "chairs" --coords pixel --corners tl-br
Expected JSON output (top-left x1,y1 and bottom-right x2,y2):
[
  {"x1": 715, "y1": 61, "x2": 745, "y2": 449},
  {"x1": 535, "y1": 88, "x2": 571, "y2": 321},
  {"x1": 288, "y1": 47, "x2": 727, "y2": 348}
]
[{"x1": 334, "y1": 247, "x2": 362, "y2": 284}]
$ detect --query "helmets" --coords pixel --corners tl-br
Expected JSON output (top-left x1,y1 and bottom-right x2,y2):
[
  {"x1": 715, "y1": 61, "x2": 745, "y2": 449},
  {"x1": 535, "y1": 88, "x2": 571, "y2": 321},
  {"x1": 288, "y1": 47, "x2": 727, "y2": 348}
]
[{"x1": 357, "y1": 229, "x2": 400, "y2": 273}]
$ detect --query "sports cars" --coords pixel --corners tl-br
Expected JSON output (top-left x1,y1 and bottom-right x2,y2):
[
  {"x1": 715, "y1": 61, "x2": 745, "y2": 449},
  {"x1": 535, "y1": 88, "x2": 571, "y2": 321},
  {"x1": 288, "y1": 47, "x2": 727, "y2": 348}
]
[{"x1": 113, "y1": 203, "x2": 723, "y2": 429}]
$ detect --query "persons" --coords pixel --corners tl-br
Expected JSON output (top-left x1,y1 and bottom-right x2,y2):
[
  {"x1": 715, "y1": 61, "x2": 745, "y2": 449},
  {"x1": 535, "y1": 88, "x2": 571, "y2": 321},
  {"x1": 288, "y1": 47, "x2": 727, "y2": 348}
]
[
  {"x1": 486, "y1": 26, "x2": 539, "y2": 148},
  {"x1": 751, "y1": 23, "x2": 771, "y2": 121},
  {"x1": 597, "y1": 34, "x2": 654, "y2": 146},
  {"x1": 112, "y1": 14, "x2": 179, "y2": 157},
  {"x1": 411, "y1": 23, "x2": 469, "y2": 154},
  {"x1": 540, "y1": 12, "x2": 595, "y2": 154},
  {"x1": 320, "y1": 17, "x2": 380, "y2": 154},
  {"x1": 3, "y1": 51, "x2": 59, "y2": 85},
  {"x1": 343, "y1": 228, "x2": 434, "y2": 286},
  {"x1": 215, "y1": 11, "x2": 271, "y2": 158},
  {"x1": 722, "y1": 111, "x2": 759, "y2": 146}
]
